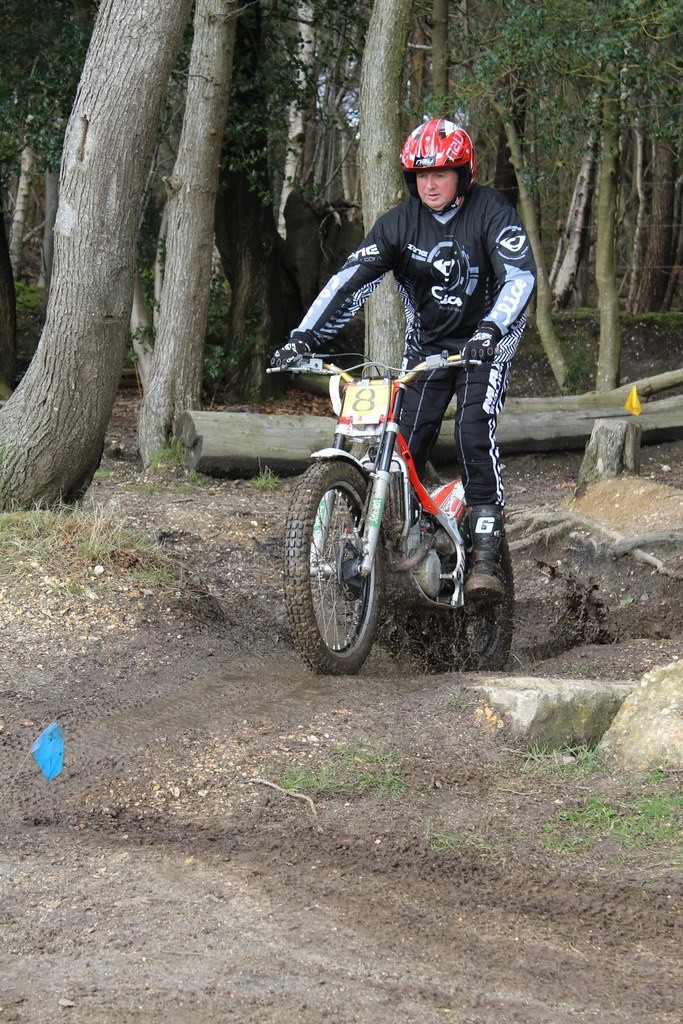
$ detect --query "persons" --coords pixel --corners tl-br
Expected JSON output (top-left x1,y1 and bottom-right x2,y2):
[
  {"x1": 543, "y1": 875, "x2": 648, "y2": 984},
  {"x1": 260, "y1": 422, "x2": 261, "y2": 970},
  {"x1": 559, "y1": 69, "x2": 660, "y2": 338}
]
[{"x1": 269, "y1": 118, "x2": 537, "y2": 601}]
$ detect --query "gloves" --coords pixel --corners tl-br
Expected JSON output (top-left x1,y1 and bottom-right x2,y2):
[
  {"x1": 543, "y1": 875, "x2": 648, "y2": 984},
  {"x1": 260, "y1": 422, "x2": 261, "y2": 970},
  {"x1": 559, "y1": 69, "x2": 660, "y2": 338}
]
[
  {"x1": 270, "y1": 331, "x2": 317, "y2": 372},
  {"x1": 461, "y1": 321, "x2": 502, "y2": 373}
]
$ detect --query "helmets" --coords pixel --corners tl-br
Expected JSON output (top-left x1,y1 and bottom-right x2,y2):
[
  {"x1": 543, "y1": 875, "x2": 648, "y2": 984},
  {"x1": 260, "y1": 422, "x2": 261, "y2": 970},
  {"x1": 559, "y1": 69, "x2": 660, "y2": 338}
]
[{"x1": 400, "y1": 118, "x2": 477, "y2": 198}]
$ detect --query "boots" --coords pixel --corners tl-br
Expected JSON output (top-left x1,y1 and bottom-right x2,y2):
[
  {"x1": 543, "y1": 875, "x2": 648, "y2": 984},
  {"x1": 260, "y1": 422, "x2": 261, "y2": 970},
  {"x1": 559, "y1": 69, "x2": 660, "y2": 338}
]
[{"x1": 464, "y1": 504, "x2": 506, "y2": 601}]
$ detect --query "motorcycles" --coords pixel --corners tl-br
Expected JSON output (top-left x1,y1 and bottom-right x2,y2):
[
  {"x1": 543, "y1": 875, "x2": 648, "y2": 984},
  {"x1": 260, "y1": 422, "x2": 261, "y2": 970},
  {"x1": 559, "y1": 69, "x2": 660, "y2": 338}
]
[{"x1": 264, "y1": 344, "x2": 513, "y2": 675}]
